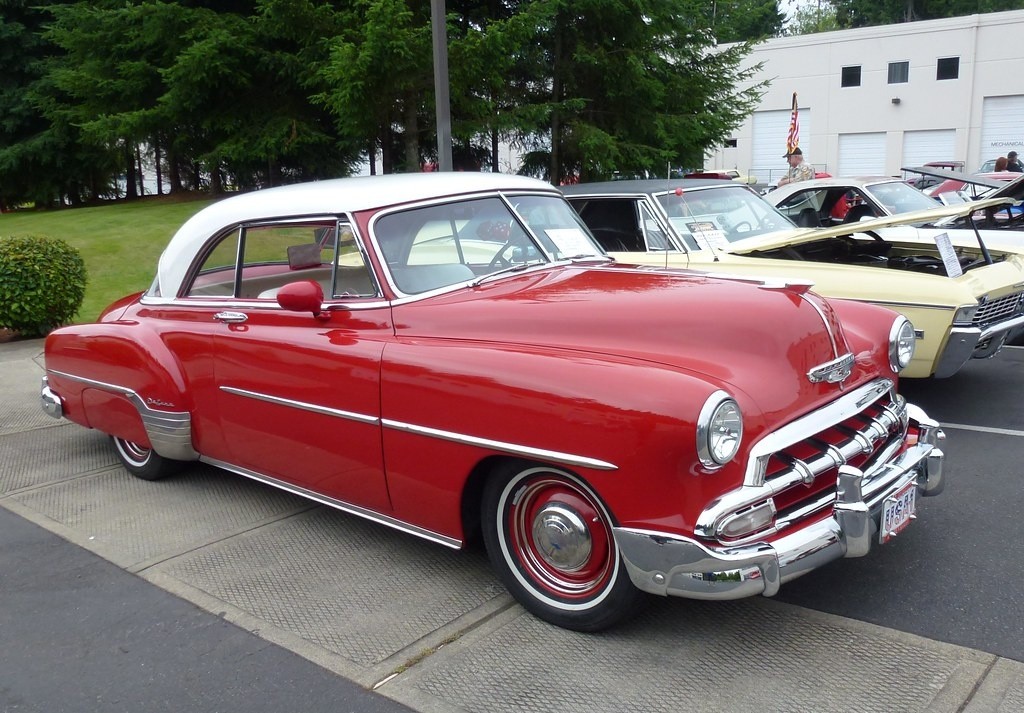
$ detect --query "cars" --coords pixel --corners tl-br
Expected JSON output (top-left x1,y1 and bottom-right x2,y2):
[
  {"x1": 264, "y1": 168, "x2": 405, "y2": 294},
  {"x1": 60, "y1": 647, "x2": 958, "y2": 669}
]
[
  {"x1": 40, "y1": 170, "x2": 949, "y2": 633},
  {"x1": 918, "y1": 172, "x2": 1024, "y2": 217},
  {"x1": 704, "y1": 169, "x2": 758, "y2": 187},
  {"x1": 639, "y1": 175, "x2": 1024, "y2": 271},
  {"x1": 339, "y1": 178, "x2": 1024, "y2": 379}
]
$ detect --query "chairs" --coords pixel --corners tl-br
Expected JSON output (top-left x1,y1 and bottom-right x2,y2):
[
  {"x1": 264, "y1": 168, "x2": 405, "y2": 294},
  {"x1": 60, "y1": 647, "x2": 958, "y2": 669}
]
[
  {"x1": 839, "y1": 203, "x2": 875, "y2": 223},
  {"x1": 256, "y1": 276, "x2": 359, "y2": 299},
  {"x1": 393, "y1": 263, "x2": 476, "y2": 294},
  {"x1": 796, "y1": 207, "x2": 823, "y2": 228}
]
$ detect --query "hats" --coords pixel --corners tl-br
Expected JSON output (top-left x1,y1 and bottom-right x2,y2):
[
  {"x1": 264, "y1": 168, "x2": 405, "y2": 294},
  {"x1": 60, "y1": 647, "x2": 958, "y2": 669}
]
[
  {"x1": 1008, "y1": 151, "x2": 1019, "y2": 158},
  {"x1": 783, "y1": 147, "x2": 802, "y2": 158}
]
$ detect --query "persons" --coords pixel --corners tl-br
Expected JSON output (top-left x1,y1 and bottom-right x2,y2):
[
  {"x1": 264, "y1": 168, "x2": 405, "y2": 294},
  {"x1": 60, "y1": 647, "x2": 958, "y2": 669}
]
[
  {"x1": 993, "y1": 156, "x2": 1009, "y2": 172},
  {"x1": 1006, "y1": 151, "x2": 1024, "y2": 172},
  {"x1": 778, "y1": 145, "x2": 816, "y2": 187}
]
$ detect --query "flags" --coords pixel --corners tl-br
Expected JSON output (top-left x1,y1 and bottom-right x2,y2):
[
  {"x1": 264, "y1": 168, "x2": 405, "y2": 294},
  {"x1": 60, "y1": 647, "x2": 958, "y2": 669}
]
[{"x1": 787, "y1": 93, "x2": 800, "y2": 154}]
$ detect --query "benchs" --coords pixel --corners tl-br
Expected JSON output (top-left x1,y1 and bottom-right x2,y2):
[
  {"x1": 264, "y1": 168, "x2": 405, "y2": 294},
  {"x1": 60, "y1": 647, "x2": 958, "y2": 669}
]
[{"x1": 187, "y1": 267, "x2": 344, "y2": 299}]
